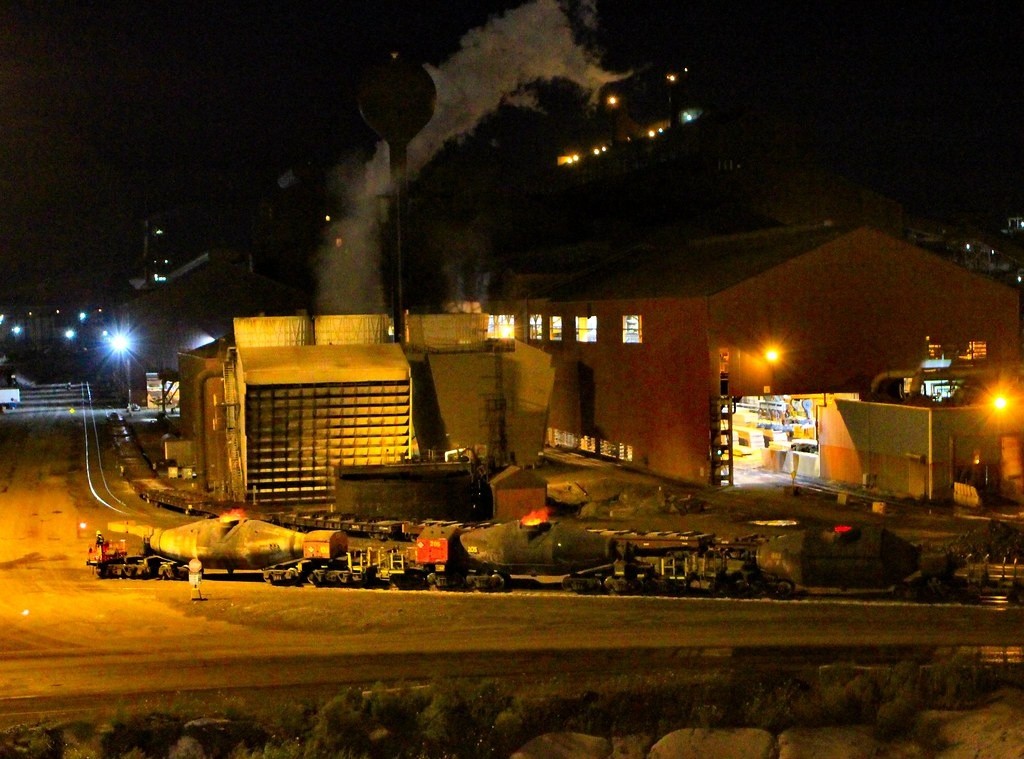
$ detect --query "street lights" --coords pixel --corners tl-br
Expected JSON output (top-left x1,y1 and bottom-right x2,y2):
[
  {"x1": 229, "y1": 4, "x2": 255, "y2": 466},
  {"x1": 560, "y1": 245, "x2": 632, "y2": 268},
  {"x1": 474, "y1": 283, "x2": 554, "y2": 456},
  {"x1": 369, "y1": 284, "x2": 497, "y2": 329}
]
[{"x1": 113, "y1": 336, "x2": 131, "y2": 412}]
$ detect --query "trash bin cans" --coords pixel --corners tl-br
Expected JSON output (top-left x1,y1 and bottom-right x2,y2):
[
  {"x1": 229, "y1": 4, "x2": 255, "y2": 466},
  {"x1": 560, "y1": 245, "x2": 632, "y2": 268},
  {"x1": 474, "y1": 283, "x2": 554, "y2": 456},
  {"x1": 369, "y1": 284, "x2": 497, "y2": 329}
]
[
  {"x1": 183, "y1": 468, "x2": 193, "y2": 480},
  {"x1": 169, "y1": 467, "x2": 179, "y2": 479}
]
